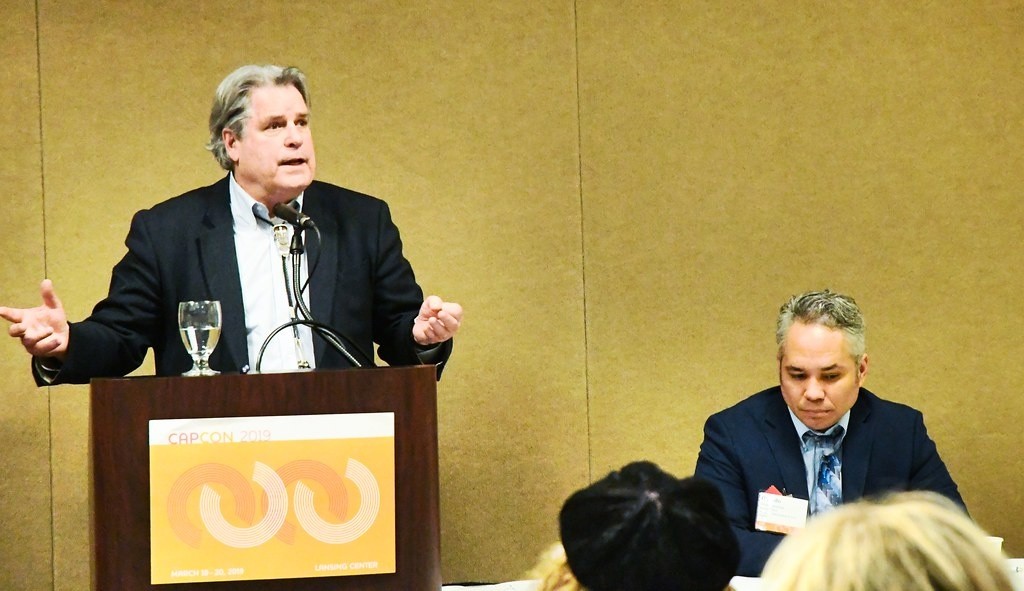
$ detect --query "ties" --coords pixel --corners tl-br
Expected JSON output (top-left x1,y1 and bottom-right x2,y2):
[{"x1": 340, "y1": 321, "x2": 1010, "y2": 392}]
[{"x1": 804, "y1": 425, "x2": 844, "y2": 515}]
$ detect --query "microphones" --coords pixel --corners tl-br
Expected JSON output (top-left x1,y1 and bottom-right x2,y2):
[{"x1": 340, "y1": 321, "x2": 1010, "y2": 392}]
[{"x1": 272, "y1": 201, "x2": 315, "y2": 229}]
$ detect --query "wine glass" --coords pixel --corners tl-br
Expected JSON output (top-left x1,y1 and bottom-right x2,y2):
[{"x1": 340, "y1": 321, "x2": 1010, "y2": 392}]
[{"x1": 177, "y1": 300, "x2": 222, "y2": 376}]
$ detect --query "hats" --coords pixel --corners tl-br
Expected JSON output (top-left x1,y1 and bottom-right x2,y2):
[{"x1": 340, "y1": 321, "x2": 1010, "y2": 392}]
[{"x1": 560, "y1": 461, "x2": 740, "y2": 591}]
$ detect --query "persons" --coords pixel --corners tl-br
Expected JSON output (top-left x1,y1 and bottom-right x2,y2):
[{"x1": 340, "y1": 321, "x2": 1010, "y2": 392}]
[
  {"x1": 0, "y1": 64, "x2": 464, "y2": 387},
  {"x1": 555, "y1": 289, "x2": 1024, "y2": 591}
]
